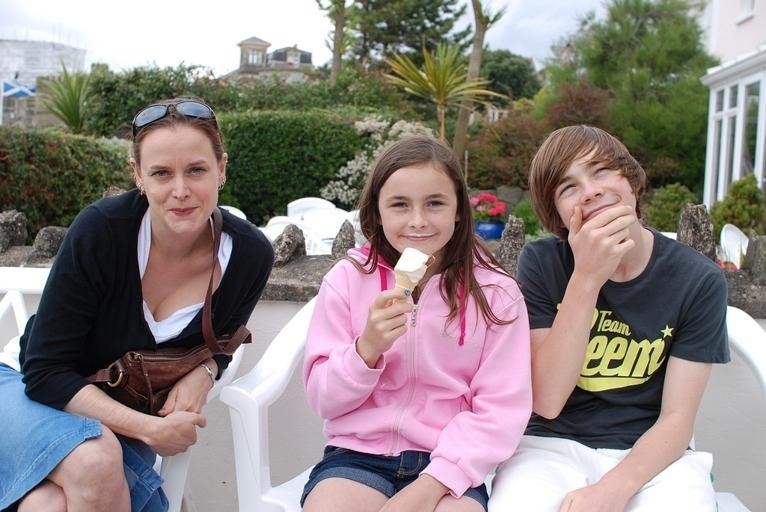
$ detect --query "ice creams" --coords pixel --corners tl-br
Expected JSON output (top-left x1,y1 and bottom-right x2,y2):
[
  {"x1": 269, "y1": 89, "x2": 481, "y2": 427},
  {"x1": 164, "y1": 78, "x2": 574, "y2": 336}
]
[{"x1": 385, "y1": 248, "x2": 429, "y2": 305}]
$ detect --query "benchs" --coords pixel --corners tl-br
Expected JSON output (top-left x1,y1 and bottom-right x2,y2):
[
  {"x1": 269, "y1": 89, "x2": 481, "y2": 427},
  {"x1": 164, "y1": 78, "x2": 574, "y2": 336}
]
[{"x1": 226, "y1": 294, "x2": 766, "y2": 512}]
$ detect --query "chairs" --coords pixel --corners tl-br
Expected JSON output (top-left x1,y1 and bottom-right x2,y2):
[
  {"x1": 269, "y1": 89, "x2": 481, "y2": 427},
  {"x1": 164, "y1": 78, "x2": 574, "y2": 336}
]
[
  {"x1": 221, "y1": 196, "x2": 363, "y2": 255},
  {"x1": 0, "y1": 264, "x2": 246, "y2": 511}
]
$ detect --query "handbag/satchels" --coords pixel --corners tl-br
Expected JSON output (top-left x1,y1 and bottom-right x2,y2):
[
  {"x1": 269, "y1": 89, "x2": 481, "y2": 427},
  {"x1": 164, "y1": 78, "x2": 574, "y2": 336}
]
[{"x1": 106, "y1": 336, "x2": 237, "y2": 444}]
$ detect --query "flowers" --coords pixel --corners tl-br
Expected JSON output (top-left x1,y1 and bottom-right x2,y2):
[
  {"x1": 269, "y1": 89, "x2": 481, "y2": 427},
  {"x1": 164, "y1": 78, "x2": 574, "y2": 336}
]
[{"x1": 469, "y1": 194, "x2": 506, "y2": 223}]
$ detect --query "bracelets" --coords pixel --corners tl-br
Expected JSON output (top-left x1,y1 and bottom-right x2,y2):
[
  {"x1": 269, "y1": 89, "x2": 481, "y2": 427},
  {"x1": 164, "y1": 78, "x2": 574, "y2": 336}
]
[{"x1": 199, "y1": 362, "x2": 216, "y2": 387}]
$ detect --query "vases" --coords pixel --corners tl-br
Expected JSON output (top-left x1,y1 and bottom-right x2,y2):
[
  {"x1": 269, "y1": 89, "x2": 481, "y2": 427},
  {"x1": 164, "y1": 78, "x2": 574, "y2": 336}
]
[{"x1": 475, "y1": 222, "x2": 504, "y2": 241}]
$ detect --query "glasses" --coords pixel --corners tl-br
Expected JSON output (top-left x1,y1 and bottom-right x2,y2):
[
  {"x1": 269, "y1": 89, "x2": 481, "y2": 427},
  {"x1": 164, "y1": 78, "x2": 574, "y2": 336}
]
[{"x1": 133, "y1": 101, "x2": 218, "y2": 139}]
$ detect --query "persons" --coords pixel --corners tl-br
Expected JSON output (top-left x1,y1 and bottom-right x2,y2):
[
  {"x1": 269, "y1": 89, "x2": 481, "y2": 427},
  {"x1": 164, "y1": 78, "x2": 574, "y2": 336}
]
[
  {"x1": 1, "y1": 98, "x2": 274, "y2": 512},
  {"x1": 487, "y1": 125, "x2": 730, "y2": 512},
  {"x1": 300, "y1": 139, "x2": 533, "y2": 512}
]
